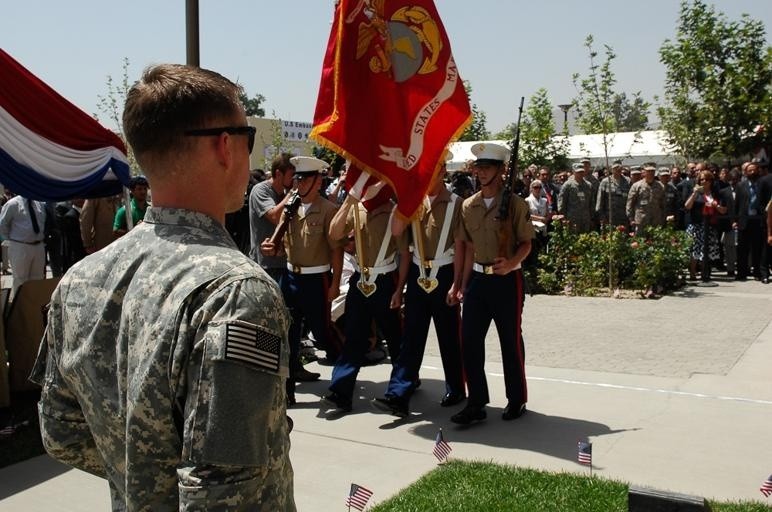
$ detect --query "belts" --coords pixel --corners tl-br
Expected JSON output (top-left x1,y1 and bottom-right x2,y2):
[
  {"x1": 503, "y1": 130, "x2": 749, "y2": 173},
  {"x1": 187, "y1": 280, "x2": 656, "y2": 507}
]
[
  {"x1": 286, "y1": 262, "x2": 330, "y2": 275},
  {"x1": 413, "y1": 255, "x2": 454, "y2": 269},
  {"x1": 354, "y1": 263, "x2": 397, "y2": 275},
  {"x1": 472, "y1": 263, "x2": 521, "y2": 275},
  {"x1": 10, "y1": 239, "x2": 40, "y2": 244}
]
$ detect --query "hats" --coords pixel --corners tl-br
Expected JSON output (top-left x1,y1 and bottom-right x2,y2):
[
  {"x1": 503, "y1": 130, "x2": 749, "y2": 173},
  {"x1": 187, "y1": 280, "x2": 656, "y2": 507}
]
[
  {"x1": 289, "y1": 156, "x2": 330, "y2": 179},
  {"x1": 471, "y1": 143, "x2": 510, "y2": 166},
  {"x1": 572, "y1": 158, "x2": 670, "y2": 176}
]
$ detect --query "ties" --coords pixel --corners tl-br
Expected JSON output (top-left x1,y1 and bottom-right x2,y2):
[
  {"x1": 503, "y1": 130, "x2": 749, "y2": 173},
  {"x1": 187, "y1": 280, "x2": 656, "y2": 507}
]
[{"x1": 28, "y1": 199, "x2": 39, "y2": 233}]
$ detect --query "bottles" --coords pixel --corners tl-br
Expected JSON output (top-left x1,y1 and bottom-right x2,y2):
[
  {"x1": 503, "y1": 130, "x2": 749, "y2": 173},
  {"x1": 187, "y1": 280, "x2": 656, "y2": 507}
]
[
  {"x1": 548, "y1": 204, "x2": 553, "y2": 213},
  {"x1": 734, "y1": 227, "x2": 738, "y2": 246}
]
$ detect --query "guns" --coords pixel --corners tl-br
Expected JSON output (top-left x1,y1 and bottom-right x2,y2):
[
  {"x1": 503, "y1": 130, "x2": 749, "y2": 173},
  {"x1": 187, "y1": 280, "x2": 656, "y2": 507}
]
[
  {"x1": 268, "y1": 146, "x2": 329, "y2": 255},
  {"x1": 494, "y1": 96, "x2": 526, "y2": 262}
]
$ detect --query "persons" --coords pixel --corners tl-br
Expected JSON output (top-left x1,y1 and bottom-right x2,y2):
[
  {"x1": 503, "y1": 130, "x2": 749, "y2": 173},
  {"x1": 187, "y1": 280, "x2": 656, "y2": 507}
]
[
  {"x1": 28, "y1": 64, "x2": 297, "y2": 512},
  {"x1": 536, "y1": 158, "x2": 772, "y2": 284},
  {"x1": 0, "y1": 175, "x2": 150, "y2": 298},
  {"x1": 250, "y1": 142, "x2": 537, "y2": 425}
]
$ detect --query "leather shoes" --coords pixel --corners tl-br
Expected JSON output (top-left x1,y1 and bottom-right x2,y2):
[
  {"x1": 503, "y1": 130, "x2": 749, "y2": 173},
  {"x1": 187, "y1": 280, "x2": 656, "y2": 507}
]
[{"x1": 286, "y1": 371, "x2": 526, "y2": 423}]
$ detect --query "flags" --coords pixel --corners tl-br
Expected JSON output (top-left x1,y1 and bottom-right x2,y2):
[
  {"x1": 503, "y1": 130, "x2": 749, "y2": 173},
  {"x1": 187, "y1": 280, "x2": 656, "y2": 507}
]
[
  {"x1": 578, "y1": 441, "x2": 592, "y2": 463},
  {"x1": 432, "y1": 430, "x2": 452, "y2": 462},
  {"x1": 345, "y1": 483, "x2": 374, "y2": 512},
  {"x1": 760, "y1": 474, "x2": 772, "y2": 498},
  {"x1": 308, "y1": 0, "x2": 473, "y2": 223}
]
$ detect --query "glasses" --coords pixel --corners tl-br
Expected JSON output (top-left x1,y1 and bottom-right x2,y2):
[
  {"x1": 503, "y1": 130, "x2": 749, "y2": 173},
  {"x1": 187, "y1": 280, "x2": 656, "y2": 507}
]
[{"x1": 184, "y1": 126, "x2": 256, "y2": 153}]
